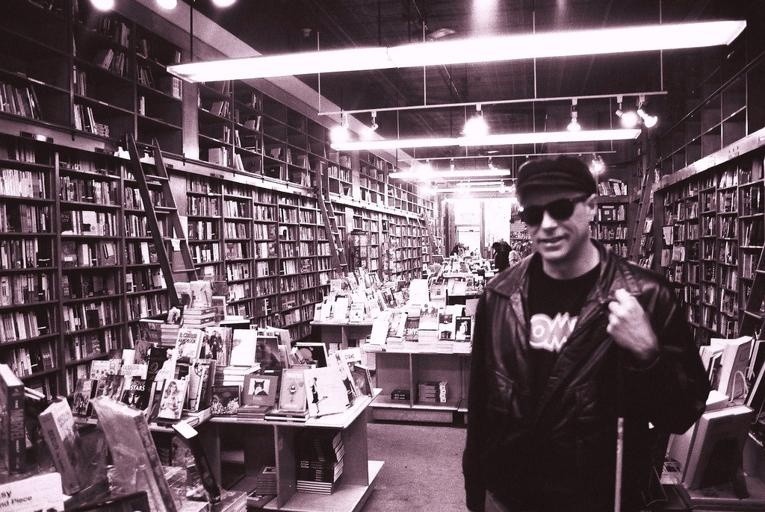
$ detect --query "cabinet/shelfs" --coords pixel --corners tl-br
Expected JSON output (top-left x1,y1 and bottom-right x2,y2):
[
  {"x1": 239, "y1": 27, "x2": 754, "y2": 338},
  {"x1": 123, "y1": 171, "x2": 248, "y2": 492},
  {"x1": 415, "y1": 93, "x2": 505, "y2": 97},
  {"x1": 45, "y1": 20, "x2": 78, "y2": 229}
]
[
  {"x1": 508, "y1": 37, "x2": 765, "y2": 511},
  {"x1": 447, "y1": 258, "x2": 501, "y2": 426},
  {"x1": 0, "y1": 1, "x2": 446, "y2": 512}
]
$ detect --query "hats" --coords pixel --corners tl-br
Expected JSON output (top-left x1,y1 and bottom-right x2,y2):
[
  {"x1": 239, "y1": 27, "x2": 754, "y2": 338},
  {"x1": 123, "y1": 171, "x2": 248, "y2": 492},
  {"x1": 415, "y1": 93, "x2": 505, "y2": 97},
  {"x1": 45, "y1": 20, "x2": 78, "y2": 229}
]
[{"x1": 515, "y1": 156, "x2": 596, "y2": 192}]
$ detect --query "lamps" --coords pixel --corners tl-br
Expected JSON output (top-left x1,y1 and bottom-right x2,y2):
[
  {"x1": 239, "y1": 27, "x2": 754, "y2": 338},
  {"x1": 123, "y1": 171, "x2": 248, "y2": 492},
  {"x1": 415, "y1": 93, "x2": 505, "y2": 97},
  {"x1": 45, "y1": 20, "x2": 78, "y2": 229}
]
[
  {"x1": 313, "y1": 0, "x2": 666, "y2": 141},
  {"x1": 166, "y1": 0, "x2": 748, "y2": 86},
  {"x1": 397, "y1": 97, "x2": 617, "y2": 172},
  {"x1": 329, "y1": 79, "x2": 642, "y2": 153}
]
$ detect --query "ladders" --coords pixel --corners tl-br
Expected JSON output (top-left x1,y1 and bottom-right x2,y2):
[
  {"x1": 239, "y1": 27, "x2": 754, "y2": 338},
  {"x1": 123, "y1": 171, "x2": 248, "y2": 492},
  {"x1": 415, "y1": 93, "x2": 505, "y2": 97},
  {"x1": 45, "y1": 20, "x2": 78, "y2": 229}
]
[
  {"x1": 314, "y1": 188, "x2": 350, "y2": 273},
  {"x1": 625, "y1": 141, "x2": 660, "y2": 263},
  {"x1": 422, "y1": 212, "x2": 443, "y2": 255},
  {"x1": 125, "y1": 130, "x2": 203, "y2": 307}
]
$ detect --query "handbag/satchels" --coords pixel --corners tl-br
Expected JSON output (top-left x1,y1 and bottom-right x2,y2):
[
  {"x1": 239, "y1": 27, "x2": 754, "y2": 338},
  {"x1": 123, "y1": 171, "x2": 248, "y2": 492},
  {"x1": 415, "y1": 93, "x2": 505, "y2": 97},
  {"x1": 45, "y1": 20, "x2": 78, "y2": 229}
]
[{"x1": 643, "y1": 482, "x2": 692, "y2": 512}]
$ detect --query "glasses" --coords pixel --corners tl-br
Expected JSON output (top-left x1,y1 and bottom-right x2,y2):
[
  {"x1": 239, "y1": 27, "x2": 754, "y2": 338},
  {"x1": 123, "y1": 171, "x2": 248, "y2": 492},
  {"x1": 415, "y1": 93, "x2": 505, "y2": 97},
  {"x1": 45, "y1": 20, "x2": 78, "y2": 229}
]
[{"x1": 518, "y1": 196, "x2": 587, "y2": 223}]
